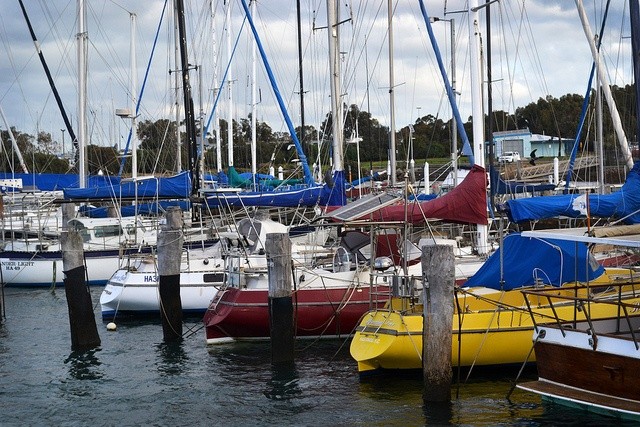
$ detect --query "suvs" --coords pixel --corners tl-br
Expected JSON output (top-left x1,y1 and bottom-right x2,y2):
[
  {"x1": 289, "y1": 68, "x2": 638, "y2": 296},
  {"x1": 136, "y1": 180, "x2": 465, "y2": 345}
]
[{"x1": 498, "y1": 151, "x2": 520, "y2": 162}]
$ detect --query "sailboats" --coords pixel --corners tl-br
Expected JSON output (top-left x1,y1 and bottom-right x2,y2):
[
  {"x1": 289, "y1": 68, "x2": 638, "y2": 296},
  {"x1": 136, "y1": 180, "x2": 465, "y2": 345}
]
[
  {"x1": 349, "y1": 0, "x2": 640, "y2": 372},
  {"x1": 202, "y1": 0, "x2": 640, "y2": 346},
  {"x1": 516, "y1": 1, "x2": 640, "y2": 421},
  {"x1": 100, "y1": 0, "x2": 499, "y2": 323}
]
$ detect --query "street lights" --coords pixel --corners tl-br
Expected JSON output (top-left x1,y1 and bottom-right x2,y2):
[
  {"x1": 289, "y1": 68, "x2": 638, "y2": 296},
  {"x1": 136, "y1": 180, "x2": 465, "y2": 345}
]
[{"x1": 428, "y1": 17, "x2": 458, "y2": 191}]
